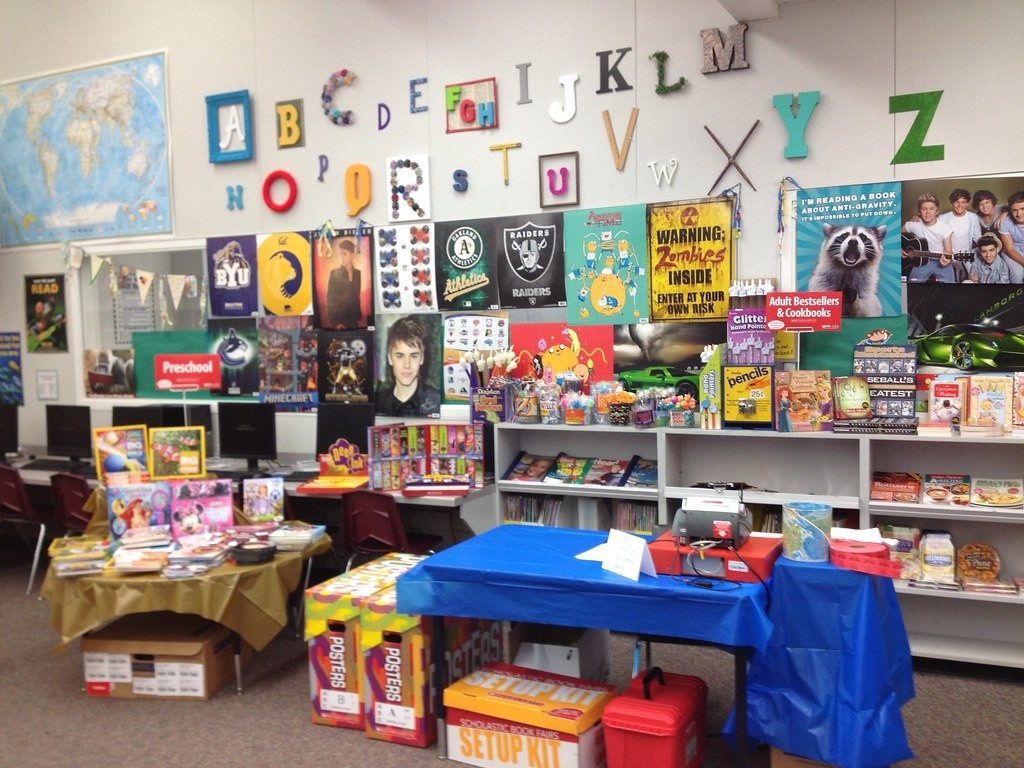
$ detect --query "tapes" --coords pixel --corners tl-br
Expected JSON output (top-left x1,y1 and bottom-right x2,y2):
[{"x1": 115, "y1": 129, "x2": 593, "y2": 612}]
[{"x1": 231, "y1": 542, "x2": 276, "y2": 565}]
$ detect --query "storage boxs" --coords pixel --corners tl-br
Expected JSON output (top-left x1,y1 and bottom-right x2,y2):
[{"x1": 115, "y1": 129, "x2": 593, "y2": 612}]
[
  {"x1": 600, "y1": 667, "x2": 708, "y2": 768},
  {"x1": 771, "y1": 745, "x2": 838, "y2": 768},
  {"x1": 443, "y1": 661, "x2": 618, "y2": 768},
  {"x1": 303, "y1": 552, "x2": 430, "y2": 730},
  {"x1": 359, "y1": 587, "x2": 518, "y2": 747},
  {"x1": 81, "y1": 610, "x2": 251, "y2": 700},
  {"x1": 512, "y1": 627, "x2": 608, "y2": 684}
]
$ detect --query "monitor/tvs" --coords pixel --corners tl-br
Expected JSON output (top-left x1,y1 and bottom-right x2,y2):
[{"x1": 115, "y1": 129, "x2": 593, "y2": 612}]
[{"x1": 0, "y1": 403, "x2": 374, "y2": 473}]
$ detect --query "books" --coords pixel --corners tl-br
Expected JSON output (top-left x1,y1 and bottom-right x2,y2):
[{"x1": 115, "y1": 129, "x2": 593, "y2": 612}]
[
  {"x1": 400, "y1": 451, "x2": 661, "y2": 534},
  {"x1": 725, "y1": 309, "x2": 1024, "y2": 437},
  {"x1": 749, "y1": 472, "x2": 1024, "y2": 596},
  {"x1": 48, "y1": 425, "x2": 328, "y2": 580}
]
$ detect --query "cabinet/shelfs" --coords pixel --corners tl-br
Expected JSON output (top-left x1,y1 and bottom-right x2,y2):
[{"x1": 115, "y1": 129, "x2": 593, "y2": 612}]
[{"x1": 494, "y1": 423, "x2": 1024, "y2": 669}]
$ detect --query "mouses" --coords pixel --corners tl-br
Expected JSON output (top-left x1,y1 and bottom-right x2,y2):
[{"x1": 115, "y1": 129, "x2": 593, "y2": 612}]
[{"x1": 252, "y1": 473, "x2": 271, "y2": 478}]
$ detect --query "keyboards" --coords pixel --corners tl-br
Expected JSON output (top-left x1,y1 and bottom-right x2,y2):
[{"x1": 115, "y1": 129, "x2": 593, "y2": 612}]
[
  {"x1": 284, "y1": 471, "x2": 319, "y2": 481},
  {"x1": 70, "y1": 466, "x2": 97, "y2": 479},
  {"x1": 20, "y1": 459, "x2": 92, "y2": 472},
  {"x1": 206, "y1": 470, "x2": 252, "y2": 482}
]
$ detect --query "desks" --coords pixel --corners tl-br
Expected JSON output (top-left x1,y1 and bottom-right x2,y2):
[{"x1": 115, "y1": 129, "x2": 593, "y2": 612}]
[
  {"x1": 10, "y1": 455, "x2": 496, "y2": 535},
  {"x1": 51, "y1": 520, "x2": 331, "y2": 696},
  {"x1": 421, "y1": 524, "x2": 773, "y2": 768}
]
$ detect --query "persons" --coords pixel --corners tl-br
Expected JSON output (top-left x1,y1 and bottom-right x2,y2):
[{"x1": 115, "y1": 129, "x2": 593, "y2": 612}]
[
  {"x1": 901, "y1": 189, "x2": 1024, "y2": 284},
  {"x1": 376, "y1": 317, "x2": 440, "y2": 420},
  {"x1": 327, "y1": 241, "x2": 364, "y2": 330},
  {"x1": 512, "y1": 458, "x2": 555, "y2": 486}
]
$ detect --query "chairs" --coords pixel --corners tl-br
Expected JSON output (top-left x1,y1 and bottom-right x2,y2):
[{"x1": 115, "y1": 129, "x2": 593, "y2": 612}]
[
  {"x1": 342, "y1": 489, "x2": 442, "y2": 574},
  {"x1": 0, "y1": 465, "x2": 47, "y2": 595},
  {"x1": 38, "y1": 471, "x2": 94, "y2": 600}
]
